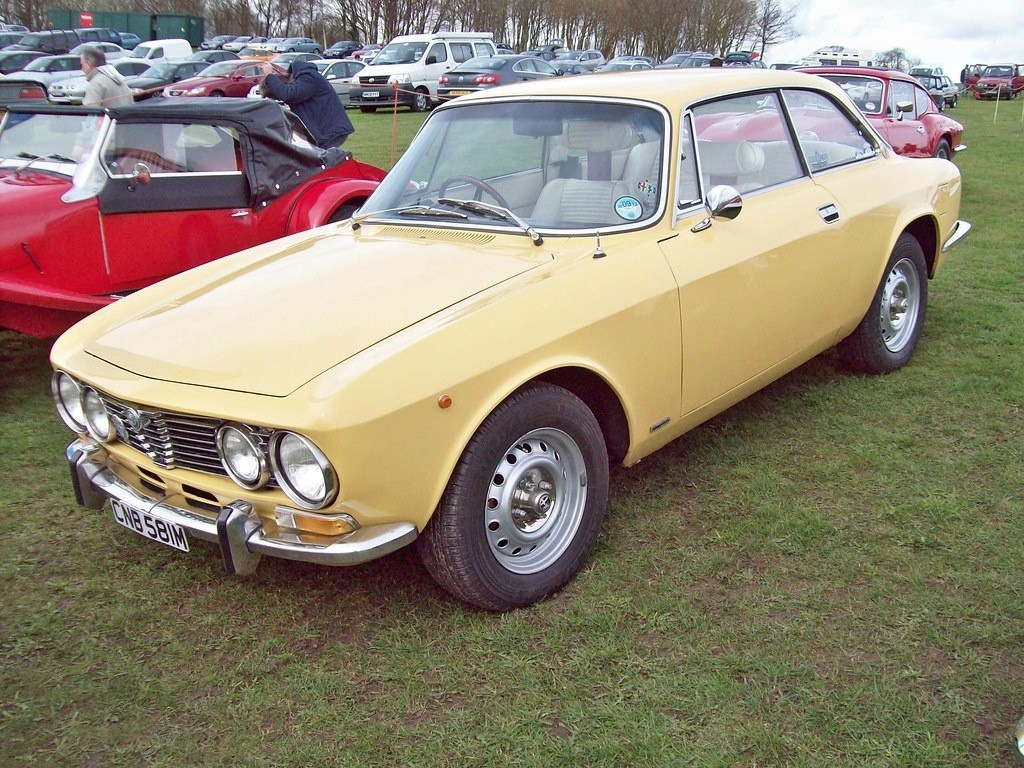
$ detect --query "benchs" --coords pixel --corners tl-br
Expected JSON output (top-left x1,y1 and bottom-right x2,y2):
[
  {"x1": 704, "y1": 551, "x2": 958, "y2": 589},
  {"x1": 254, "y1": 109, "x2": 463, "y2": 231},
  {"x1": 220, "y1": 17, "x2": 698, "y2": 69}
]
[{"x1": 623, "y1": 136, "x2": 860, "y2": 196}]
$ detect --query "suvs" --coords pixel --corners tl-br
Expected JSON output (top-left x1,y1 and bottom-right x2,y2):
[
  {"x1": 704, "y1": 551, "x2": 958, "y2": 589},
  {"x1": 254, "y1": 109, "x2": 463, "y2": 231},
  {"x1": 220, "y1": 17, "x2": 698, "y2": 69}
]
[{"x1": 0, "y1": 27, "x2": 85, "y2": 74}]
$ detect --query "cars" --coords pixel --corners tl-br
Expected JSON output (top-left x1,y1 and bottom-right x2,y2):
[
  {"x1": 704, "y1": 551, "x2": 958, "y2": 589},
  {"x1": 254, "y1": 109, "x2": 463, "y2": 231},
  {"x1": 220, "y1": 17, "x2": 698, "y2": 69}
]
[
  {"x1": 436, "y1": 53, "x2": 567, "y2": 101},
  {"x1": 185, "y1": 50, "x2": 242, "y2": 66},
  {"x1": 109, "y1": 32, "x2": 141, "y2": 52},
  {"x1": 69, "y1": 40, "x2": 133, "y2": 60},
  {"x1": 247, "y1": 59, "x2": 368, "y2": 110},
  {"x1": 966, "y1": 62, "x2": 1024, "y2": 100},
  {"x1": 237, "y1": 47, "x2": 283, "y2": 62},
  {"x1": 684, "y1": 64, "x2": 968, "y2": 169},
  {"x1": 0, "y1": 71, "x2": 50, "y2": 113},
  {"x1": 73, "y1": 26, "x2": 122, "y2": 50},
  {"x1": 122, "y1": 61, "x2": 213, "y2": 101},
  {"x1": 46, "y1": 57, "x2": 153, "y2": 106},
  {"x1": 0, "y1": 51, "x2": 54, "y2": 76},
  {"x1": 160, "y1": 59, "x2": 291, "y2": 97},
  {"x1": 50, "y1": 67, "x2": 972, "y2": 613},
  {"x1": 270, "y1": 51, "x2": 325, "y2": 69},
  {"x1": 200, "y1": 34, "x2": 383, "y2": 60},
  {"x1": 1, "y1": 97, "x2": 434, "y2": 342},
  {"x1": 6, "y1": 55, "x2": 84, "y2": 90},
  {"x1": 518, "y1": 38, "x2": 768, "y2": 75}
]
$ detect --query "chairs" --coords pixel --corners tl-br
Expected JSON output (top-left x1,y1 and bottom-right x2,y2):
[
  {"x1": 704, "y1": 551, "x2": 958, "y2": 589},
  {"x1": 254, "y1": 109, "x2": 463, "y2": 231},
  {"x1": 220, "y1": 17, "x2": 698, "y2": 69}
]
[
  {"x1": 681, "y1": 137, "x2": 767, "y2": 204},
  {"x1": 114, "y1": 124, "x2": 180, "y2": 177},
  {"x1": 533, "y1": 116, "x2": 633, "y2": 228},
  {"x1": 190, "y1": 148, "x2": 244, "y2": 172}
]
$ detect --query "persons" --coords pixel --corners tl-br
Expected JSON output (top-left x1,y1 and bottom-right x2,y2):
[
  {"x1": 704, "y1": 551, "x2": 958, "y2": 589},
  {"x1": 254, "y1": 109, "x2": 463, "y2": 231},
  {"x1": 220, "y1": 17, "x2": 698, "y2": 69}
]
[
  {"x1": 973, "y1": 65, "x2": 983, "y2": 96},
  {"x1": 80, "y1": 48, "x2": 134, "y2": 107},
  {"x1": 259, "y1": 54, "x2": 355, "y2": 150},
  {"x1": 960, "y1": 65, "x2": 971, "y2": 95}
]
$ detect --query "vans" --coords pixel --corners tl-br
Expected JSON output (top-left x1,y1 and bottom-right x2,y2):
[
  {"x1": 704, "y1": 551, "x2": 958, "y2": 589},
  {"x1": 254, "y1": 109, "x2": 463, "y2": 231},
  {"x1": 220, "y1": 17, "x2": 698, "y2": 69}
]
[
  {"x1": 120, "y1": 39, "x2": 194, "y2": 66},
  {"x1": 348, "y1": 32, "x2": 500, "y2": 114}
]
